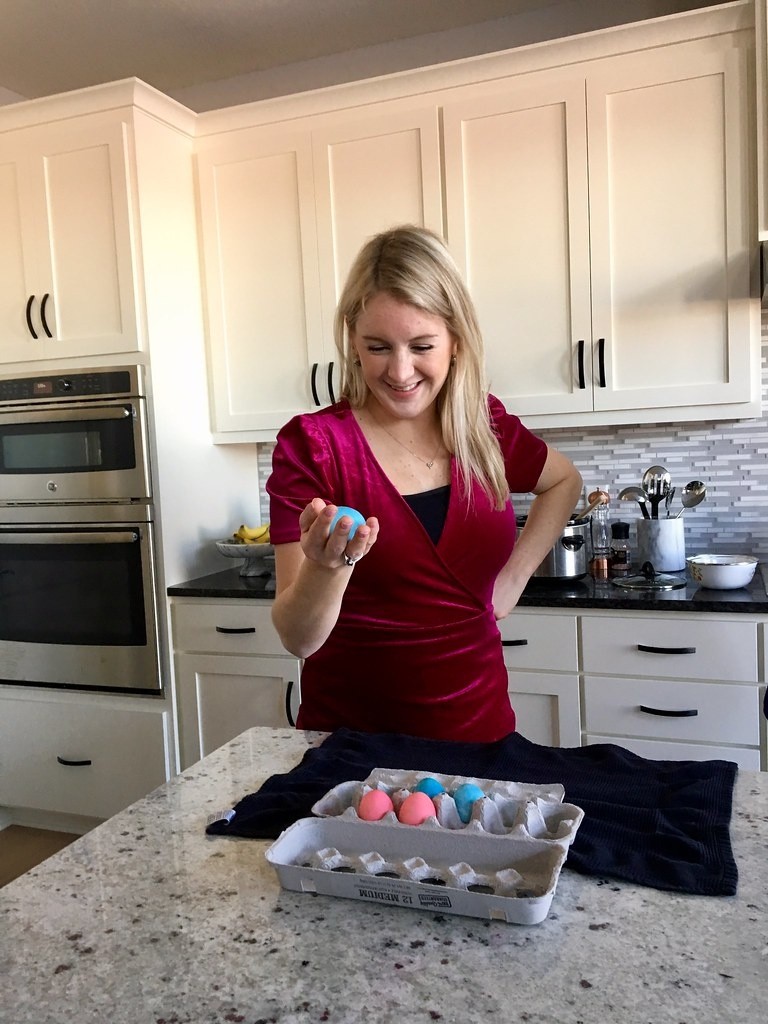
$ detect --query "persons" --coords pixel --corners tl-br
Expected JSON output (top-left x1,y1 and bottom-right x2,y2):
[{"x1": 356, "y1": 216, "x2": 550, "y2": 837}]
[{"x1": 265, "y1": 223, "x2": 582, "y2": 744}]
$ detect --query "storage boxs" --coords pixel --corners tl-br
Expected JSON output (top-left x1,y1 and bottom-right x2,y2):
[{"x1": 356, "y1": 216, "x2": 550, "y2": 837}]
[{"x1": 264, "y1": 767, "x2": 587, "y2": 924}]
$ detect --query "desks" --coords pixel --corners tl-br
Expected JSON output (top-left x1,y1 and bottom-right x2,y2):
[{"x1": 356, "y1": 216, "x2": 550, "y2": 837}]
[{"x1": 0, "y1": 725, "x2": 768, "y2": 1024}]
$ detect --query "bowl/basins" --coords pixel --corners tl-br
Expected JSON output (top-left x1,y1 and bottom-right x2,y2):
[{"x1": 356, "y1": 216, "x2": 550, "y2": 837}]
[{"x1": 686, "y1": 553, "x2": 759, "y2": 589}]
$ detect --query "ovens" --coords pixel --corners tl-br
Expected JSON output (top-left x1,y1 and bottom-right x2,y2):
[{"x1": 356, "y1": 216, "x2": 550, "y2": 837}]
[{"x1": 0, "y1": 363, "x2": 169, "y2": 696}]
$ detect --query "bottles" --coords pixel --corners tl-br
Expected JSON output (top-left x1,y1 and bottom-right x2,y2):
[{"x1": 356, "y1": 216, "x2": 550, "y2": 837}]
[
  {"x1": 592, "y1": 503, "x2": 610, "y2": 554},
  {"x1": 611, "y1": 521, "x2": 631, "y2": 570}
]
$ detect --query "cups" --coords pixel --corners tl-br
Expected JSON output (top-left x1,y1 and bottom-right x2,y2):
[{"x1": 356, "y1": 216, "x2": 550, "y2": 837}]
[{"x1": 637, "y1": 515, "x2": 686, "y2": 572}]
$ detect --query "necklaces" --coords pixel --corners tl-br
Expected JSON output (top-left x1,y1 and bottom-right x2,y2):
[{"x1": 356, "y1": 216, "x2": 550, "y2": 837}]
[{"x1": 364, "y1": 401, "x2": 442, "y2": 468}]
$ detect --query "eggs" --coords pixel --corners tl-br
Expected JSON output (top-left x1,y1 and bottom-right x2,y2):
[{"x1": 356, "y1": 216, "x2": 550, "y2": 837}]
[
  {"x1": 452, "y1": 783, "x2": 485, "y2": 823},
  {"x1": 358, "y1": 790, "x2": 393, "y2": 821},
  {"x1": 329, "y1": 506, "x2": 366, "y2": 541},
  {"x1": 413, "y1": 778, "x2": 445, "y2": 798},
  {"x1": 398, "y1": 792, "x2": 436, "y2": 825}
]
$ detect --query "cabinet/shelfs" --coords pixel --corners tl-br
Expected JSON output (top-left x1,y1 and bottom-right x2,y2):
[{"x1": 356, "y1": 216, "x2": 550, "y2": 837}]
[
  {"x1": 0, "y1": 80, "x2": 259, "y2": 842},
  {"x1": 192, "y1": 0, "x2": 768, "y2": 448},
  {"x1": 167, "y1": 552, "x2": 768, "y2": 779}
]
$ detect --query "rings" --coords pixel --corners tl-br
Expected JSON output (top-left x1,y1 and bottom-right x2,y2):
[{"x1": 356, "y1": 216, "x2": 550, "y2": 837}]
[{"x1": 344, "y1": 552, "x2": 363, "y2": 566}]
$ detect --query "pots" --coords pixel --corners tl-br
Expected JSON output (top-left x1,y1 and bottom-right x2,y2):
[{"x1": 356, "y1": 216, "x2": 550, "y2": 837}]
[{"x1": 516, "y1": 513, "x2": 590, "y2": 581}]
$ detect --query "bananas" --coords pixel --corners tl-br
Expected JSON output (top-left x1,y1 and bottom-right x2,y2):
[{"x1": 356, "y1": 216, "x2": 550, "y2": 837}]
[{"x1": 232, "y1": 523, "x2": 270, "y2": 545}]
